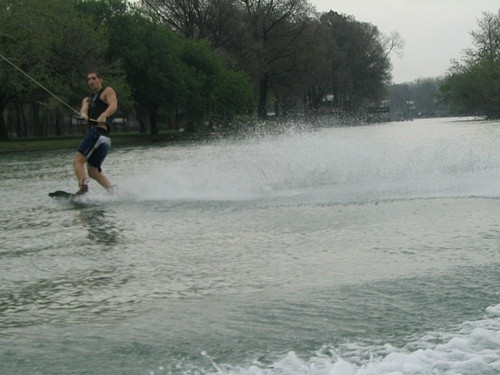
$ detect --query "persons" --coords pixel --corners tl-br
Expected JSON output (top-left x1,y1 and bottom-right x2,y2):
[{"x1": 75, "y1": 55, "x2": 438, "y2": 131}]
[{"x1": 73, "y1": 71, "x2": 118, "y2": 197}]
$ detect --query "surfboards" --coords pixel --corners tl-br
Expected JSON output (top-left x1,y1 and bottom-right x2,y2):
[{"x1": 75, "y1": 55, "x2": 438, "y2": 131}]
[{"x1": 48, "y1": 187, "x2": 119, "y2": 203}]
